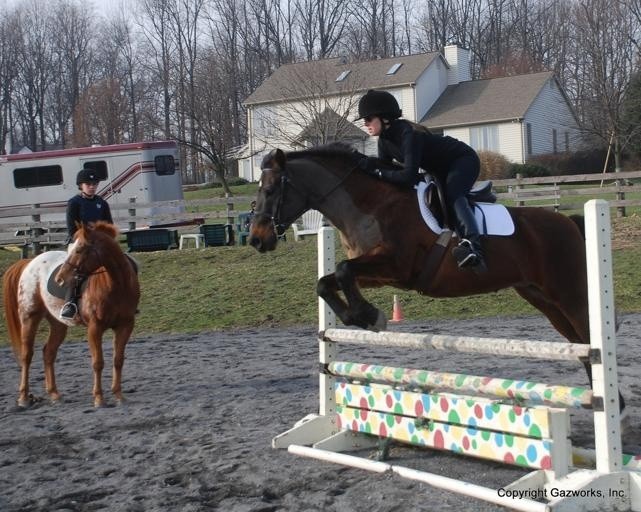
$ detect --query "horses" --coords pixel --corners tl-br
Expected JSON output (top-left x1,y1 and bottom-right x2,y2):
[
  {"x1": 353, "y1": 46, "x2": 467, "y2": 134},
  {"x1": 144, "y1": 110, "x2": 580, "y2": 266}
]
[
  {"x1": 246, "y1": 141, "x2": 625, "y2": 415},
  {"x1": 1, "y1": 218, "x2": 141, "y2": 409}
]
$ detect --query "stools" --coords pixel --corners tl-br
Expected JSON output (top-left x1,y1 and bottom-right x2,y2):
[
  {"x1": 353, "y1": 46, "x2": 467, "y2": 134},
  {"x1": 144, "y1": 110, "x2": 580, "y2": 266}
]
[{"x1": 179, "y1": 233, "x2": 206, "y2": 249}]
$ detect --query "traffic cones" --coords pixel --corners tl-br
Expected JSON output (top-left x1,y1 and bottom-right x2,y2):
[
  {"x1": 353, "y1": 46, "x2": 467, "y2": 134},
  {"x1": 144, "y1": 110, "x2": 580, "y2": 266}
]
[{"x1": 389, "y1": 294, "x2": 407, "y2": 322}]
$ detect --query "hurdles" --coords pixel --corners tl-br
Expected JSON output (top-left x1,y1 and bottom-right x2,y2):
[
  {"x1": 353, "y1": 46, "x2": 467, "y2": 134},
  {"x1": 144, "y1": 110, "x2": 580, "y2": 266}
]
[{"x1": 272, "y1": 199, "x2": 641, "y2": 512}]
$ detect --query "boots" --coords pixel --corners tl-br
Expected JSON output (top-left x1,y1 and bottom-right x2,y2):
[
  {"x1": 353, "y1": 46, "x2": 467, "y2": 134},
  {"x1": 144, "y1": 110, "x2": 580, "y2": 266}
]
[
  {"x1": 451, "y1": 197, "x2": 482, "y2": 269},
  {"x1": 61, "y1": 279, "x2": 82, "y2": 319}
]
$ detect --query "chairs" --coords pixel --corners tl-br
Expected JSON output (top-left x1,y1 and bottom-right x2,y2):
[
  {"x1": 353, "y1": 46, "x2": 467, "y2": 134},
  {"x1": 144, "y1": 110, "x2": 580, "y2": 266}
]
[
  {"x1": 293, "y1": 209, "x2": 324, "y2": 242},
  {"x1": 237, "y1": 213, "x2": 257, "y2": 245}
]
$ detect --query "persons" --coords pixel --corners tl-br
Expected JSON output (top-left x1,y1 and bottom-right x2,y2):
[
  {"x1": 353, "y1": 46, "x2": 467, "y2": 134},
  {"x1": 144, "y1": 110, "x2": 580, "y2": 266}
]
[
  {"x1": 62, "y1": 170, "x2": 140, "y2": 317},
  {"x1": 244, "y1": 201, "x2": 257, "y2": 231},
  {"x1": 353, "y1": 90, "x2": 481, "y2": 266}
]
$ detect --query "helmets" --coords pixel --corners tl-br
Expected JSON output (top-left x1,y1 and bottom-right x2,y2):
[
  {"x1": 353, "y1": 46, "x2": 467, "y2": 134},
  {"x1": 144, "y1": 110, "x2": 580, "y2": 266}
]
[
  {"x1": 352, "y1": 89, "x2": 402, "y2": 122},
  {"x1": 76, "y1": 168, "x2": 99, "y2": 185}
]
